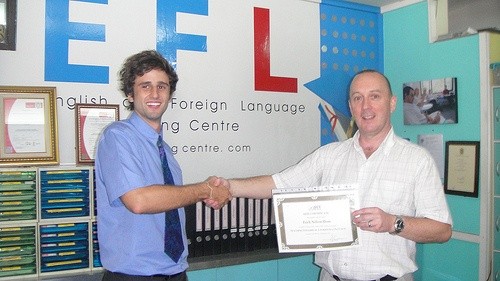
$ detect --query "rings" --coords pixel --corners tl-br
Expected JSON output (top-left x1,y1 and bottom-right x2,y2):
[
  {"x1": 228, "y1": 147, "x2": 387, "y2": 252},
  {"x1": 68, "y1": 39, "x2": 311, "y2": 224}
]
[{"x1": 368, "y1": 221, "x2": 370, "y2": 227}]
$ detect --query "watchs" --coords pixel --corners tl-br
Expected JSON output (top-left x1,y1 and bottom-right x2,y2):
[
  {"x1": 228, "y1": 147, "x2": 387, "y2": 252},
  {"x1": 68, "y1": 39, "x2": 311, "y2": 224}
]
[{"x1": 389, "y1": 215, "x2": 404, "y2": 235}]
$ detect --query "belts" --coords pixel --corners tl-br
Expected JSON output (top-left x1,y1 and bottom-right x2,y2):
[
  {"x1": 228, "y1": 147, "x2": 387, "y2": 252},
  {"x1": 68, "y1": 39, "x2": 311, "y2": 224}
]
[{"x1": 333, "y1": 274, "x2": 398, "y2": 281}]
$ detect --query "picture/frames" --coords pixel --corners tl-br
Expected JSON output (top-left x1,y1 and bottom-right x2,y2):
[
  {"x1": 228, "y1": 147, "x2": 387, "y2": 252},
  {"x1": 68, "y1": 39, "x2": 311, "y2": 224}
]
[{"x1": 0, "y1": 0, "x2": 17, "y2": 50}]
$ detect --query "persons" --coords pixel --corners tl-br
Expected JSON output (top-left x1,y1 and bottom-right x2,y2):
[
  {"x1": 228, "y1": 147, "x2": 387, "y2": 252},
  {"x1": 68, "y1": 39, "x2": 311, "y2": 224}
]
[
  {"x1": 403, "y1": 85, "x2": 450, "y2": 124},
  {"x1": 203, "y1": 70, "x2": 452, "y2": 281},
  {"x1": 95, "y1": 50, "x2": 232, "y2": 281}
]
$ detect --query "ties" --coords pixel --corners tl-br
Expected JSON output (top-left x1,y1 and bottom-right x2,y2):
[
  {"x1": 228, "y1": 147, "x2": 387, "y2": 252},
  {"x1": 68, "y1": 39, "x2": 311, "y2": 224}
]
[{"x1": 157, "y1": 135, "x2": 185, "y2": 264}]
